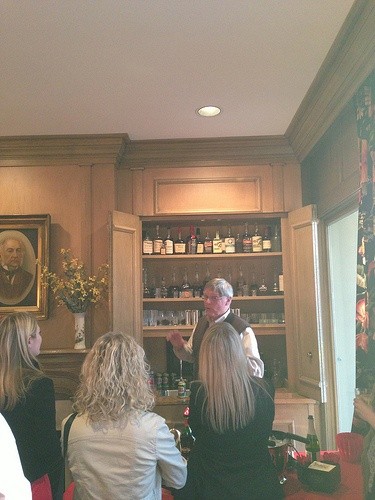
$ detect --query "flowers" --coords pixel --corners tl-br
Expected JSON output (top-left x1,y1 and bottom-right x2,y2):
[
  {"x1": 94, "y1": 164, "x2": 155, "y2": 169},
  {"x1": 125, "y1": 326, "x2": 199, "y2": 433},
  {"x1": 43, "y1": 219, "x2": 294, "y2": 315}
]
[{"x1": 37, "y1": 249, "x2": 109, "y2": 312}]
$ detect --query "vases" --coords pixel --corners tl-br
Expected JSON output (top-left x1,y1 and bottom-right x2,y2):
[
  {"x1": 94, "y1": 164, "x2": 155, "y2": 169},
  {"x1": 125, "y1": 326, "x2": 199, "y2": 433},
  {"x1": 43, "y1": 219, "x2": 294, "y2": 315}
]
[{"x1": 74, "y1": 312, "x2": 86, "y2": 350}]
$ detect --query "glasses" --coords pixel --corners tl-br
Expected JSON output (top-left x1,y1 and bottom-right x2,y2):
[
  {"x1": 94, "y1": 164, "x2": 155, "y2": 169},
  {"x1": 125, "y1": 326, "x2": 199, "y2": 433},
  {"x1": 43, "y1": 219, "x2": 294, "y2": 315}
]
[{"x1": 201, "y1": 294, "x2": 226, "y2": 303}]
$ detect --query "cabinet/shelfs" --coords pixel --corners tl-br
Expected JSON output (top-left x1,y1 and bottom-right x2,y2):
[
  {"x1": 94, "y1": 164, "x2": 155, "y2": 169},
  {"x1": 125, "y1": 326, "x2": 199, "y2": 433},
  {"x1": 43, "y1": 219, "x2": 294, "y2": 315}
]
[{"x1": 109, "y1": 204, "x2": 327, "y2": 403}]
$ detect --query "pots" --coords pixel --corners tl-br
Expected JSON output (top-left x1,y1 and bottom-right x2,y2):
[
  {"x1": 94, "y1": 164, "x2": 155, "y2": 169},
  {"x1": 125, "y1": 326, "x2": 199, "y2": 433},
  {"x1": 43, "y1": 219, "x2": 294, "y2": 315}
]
[{"x1": 267, "y1": 435, "x2": 291, "y2": 477}]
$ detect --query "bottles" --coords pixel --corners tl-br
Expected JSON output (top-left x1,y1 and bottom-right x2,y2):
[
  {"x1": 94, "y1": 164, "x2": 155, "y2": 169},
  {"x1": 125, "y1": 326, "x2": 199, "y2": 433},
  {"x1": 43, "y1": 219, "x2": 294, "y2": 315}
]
[
  {"x1": 163, "y1": 228, "x2": 174, "y2": 255},
  {"x1": 150, "y1": 370, "x2": 178, "y2": 397},
  {"x1": 231, "y1": 307, "x2": 241, "y2": 317},
  {"x1": 174, "y1": 232, "x2": 186, "y2": 254},
  {"x1": 160, "y1": 243, "x2": 166, "y2": 255},
  {"x1": 304, "y1": 415, "x2": 320, "y2": 462},
  {"x1": 177, "y1": 376, "x2": 187, "y2": 399},
  {"x1": 142, "y1": 236, "x2": 153, "y2": 255},
  {"x1": 143, "y1": 264, "x2": 284, "y2": 297},
  {"x1": 272, "y1": 224, "x2": 280, "y2": 252},
  {"x1": 153, "y1": 224, "x2": 163, "y2": 255},
  {"x1": 260, "y1": 352, "x2": 282, "y2": 386},
  {"x1": 180, "y1": 414, "x2": 194, "y2": 461},
  {"x1": 186, "y1": 222, "x2": 272, "y2": 254}
]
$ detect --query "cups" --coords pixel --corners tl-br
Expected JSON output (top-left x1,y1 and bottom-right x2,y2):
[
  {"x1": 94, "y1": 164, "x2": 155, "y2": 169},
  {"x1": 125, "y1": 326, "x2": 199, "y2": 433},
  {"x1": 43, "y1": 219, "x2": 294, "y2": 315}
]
[
  {"x1": 143, "y1": 309, "x2": 158, "y2": 326},
  {"x1": 355, "y1": 387, "x2": 372, "y2": 404},
  {"x1": 241, "y1": 312, "x2": 285, "y2": 324},
  {"x1": 185, "y1": 309, "x2": 207, "y2": 326},
  {"x1": 297, "y1": 452, "x2": 312, "y2": 464}
]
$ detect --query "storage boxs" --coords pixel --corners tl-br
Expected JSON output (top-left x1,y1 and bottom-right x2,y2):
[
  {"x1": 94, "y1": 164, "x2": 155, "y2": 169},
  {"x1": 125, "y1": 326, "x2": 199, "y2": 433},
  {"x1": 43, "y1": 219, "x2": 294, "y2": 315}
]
[{"x1": 303, "y1": 460, "x2": 340, "y2": 493}]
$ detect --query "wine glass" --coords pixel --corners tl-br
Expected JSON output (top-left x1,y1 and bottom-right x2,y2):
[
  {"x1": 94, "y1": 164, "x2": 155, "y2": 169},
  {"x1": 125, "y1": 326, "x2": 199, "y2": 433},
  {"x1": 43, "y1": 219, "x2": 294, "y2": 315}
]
[{"x1": 157, "y1": 311, "x2": 185, "y2": 326}]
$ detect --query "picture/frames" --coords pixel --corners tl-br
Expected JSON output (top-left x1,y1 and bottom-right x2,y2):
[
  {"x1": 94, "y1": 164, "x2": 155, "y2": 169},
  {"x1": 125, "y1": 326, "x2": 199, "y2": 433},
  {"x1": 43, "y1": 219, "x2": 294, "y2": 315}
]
[{"x1": 0, "y1": 214, "x2": 49, "y2": 320}]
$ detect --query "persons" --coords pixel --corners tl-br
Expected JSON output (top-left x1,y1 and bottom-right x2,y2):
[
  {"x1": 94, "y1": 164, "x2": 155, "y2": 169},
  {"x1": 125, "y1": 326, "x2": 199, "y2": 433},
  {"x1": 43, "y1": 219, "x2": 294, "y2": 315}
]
[
  {"x1": 0, "y1": 311, "x2": 65, "y2": 500},
  {"x1": 171, "y1": 322, "x2": 286, "y2": 500},
  {"x1": 353, "y1": 384, "x2": 375, "y2": 500},
  {"x1": 61, "y1": 331, "x2": 187, "y2": 500},
  {"x1": 166, "y1": 277, "x2": 264, "y2": 378},
  {"x1": 0, "y1": 236, "x2": 33, "y2": 299}
]
